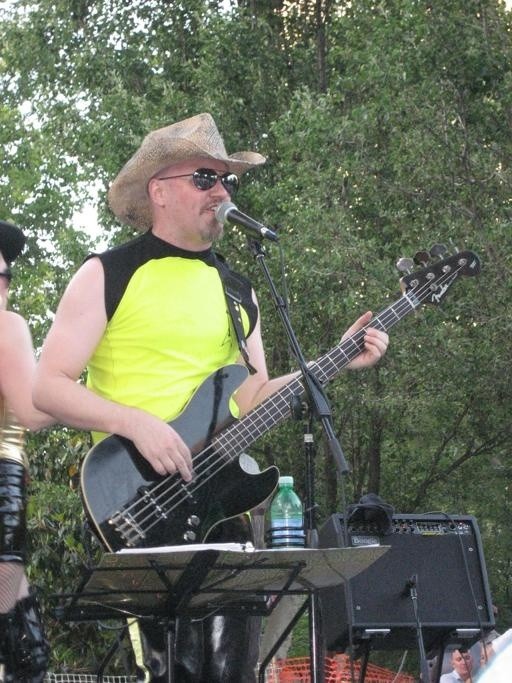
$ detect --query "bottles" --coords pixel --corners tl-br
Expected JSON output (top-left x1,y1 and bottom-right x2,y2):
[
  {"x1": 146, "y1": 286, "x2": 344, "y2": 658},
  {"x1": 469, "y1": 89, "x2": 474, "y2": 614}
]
[{"x1": 269, "y1": 475, "x2": 306, "y2": 549}]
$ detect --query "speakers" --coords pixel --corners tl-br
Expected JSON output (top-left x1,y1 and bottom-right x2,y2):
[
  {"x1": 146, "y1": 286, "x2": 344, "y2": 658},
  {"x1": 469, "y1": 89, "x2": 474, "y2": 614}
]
[{"x1": 317, "y1": 513, "x2": 496, "y2": 651}]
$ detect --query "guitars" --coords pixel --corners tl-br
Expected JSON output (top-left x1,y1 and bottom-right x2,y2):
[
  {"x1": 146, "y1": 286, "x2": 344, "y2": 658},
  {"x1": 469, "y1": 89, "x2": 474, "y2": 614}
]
[{"x1": 78, "y1": 234, "x2": 482, "y2": 554}]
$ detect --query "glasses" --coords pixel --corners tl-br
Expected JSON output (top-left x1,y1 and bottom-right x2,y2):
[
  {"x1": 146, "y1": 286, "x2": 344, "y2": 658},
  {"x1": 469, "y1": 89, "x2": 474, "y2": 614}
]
[
  {"x1": 0, "y1": 270, "x2": 12, "y2": 284},
  {"x1": 159, "y1": 169, "x2": 239, "y2": 196}
]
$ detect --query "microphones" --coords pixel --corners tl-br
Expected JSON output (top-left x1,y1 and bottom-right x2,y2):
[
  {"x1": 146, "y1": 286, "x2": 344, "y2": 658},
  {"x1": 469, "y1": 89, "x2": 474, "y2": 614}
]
[{"x1": 215, "y1": 201, "x2": 277, "y2": 241}]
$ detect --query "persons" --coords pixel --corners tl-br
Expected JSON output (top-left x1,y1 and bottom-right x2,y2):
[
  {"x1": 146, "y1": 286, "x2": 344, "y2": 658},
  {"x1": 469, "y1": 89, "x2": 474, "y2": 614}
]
[
  {"x1": 31, "y1": 113, "x2": 390, "y2": 683},
  {"x1": 1, "y1": 221, "x2": 59, "y2": 681},
  {"x1": 440, "y1": 649, "x2": 475, "y2": 683},
  {"x1": 479, "y1": 642, "x2": 494, "y2": 666}
]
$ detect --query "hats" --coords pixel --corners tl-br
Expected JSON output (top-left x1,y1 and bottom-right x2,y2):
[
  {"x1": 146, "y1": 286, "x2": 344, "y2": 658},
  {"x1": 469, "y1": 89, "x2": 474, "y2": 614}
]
[
  {"x1": 108, "y1": 112, "x2": 265, "y2": 232},
  {"x1": 0, "y1": 220, "x2": 26, "y2": 263}
]
